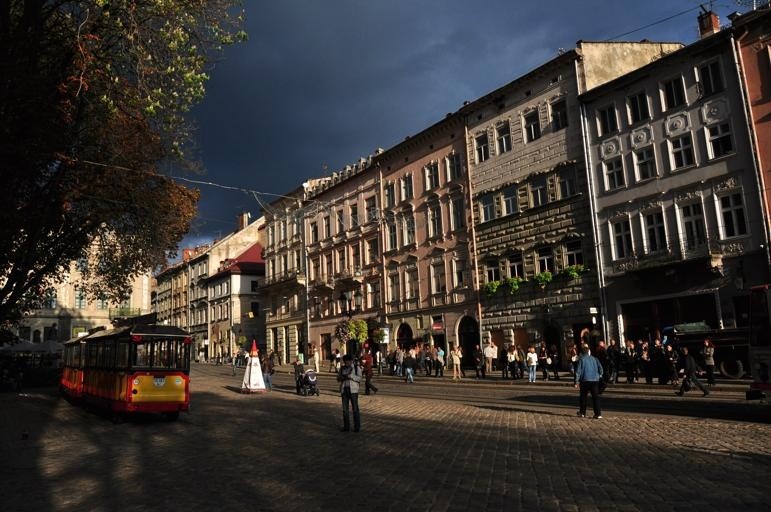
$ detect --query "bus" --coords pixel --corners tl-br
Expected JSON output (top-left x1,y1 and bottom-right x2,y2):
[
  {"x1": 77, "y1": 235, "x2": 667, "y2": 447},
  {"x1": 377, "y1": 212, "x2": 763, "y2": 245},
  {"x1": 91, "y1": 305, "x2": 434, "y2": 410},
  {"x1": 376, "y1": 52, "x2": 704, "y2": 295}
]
[
  {"x1": 746, "y1": 283, "x2": 771, "y2": 406},
  {"x1": 59, "y1": 325, "x2": 192, "y2": 422}
]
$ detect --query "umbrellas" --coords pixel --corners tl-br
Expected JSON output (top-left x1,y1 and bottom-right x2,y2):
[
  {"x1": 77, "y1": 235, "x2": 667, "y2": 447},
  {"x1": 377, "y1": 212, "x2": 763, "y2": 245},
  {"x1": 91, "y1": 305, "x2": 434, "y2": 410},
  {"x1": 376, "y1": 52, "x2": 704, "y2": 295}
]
[
  {"x1": 33, "y1": 340, "x2": 68, "y2": 365},
  {"x1": 9, "y1": 336, "x2": 35, "y2": 363}
]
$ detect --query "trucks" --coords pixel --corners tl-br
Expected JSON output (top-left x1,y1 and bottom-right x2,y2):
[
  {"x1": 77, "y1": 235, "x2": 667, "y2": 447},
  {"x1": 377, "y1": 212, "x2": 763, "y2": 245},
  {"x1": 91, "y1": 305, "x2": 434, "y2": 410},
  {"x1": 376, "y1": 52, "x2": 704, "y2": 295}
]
[{"x1": 660, "y1": 321, "x2": 748, "y2": 378}]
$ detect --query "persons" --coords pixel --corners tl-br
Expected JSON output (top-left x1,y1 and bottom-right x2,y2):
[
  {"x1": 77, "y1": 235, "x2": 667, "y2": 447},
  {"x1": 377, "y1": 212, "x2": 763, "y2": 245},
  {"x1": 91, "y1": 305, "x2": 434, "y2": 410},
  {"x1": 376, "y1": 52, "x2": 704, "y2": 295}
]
[
  {"x1": 231, "y1": 353, "x2": 238, "y2": 376},
  {"x1": 569, "y1": 338, "x2": 680, "y2": 385},
  {"x1": 237, "y1": 348, "x2": 275, "y2": 367},
  {"x1": 329, "y1": 352, "x2": 339, "y2": 372},
  {"x1": 338, "y1": 355, "x2": 365, "y2": 432},
  {"x1": 216, "y1": 353, "x2": 223, "y2": 365},
  {"x1": 703, "y1": 339, "x2": 715, "y2": 384},
  {"x1": 574, "y1": 347, "x2": 604, "y2": 418},
  {"x1": 294, "y1": 360, "x2": 306, "y2": 393},
  {"x1": 262, "y1": 354, "x2": 273, "y2": 392},
  {"x1": 674, "y1": 348, "x2": 709, "y2": 396},
  {"x1": 336, "y1": 348, "x2": 341, "y2": 367},
  {"x1": 361, "y1": 348, "x2": 378, "y2": 395},
  {"x1": 376, "y1": 350, "x2": 383, "y2": 375},
  {"x1": 386, "y1": 343, "x2": 465, "y2": 384},
  {"x1": 474, "y1": 341, "x2": 560, "y2": 383}
]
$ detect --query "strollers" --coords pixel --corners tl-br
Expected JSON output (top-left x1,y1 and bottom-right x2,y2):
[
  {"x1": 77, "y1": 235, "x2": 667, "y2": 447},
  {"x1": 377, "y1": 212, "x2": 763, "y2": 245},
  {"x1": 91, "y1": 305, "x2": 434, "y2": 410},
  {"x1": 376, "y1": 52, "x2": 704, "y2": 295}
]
[{"x1": 300, "y1": 369, "x2": 320, "y2": 397}]
